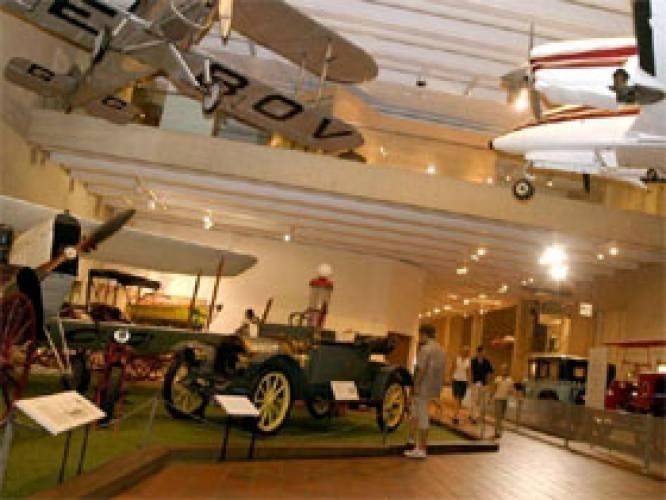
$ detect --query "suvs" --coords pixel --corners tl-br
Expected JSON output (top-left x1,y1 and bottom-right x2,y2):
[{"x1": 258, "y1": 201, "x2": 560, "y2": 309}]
[
  {"x1": 160, "y1": 297, "x2": 411, "y2": 436},
  {"x1": 514, "y1": 339, "x2": 666, "y2": 416}
]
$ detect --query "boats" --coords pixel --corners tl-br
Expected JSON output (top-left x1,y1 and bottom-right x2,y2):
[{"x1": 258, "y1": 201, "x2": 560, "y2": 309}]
[{"x1": 126, "y1": 292, "x2": 217, "y2": 327}]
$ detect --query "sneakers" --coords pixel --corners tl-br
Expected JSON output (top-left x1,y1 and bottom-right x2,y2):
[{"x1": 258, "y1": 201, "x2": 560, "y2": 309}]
[{"x1": 403, "y1": 447, "x2": 428, "y2": 458}]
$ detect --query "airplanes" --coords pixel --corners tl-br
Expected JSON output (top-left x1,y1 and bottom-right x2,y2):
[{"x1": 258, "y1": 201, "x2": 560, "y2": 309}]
[
  {"x1": 0, "y1": 0, "x2": 381, "y2": 155},
  {"x1": 497, "y1": 0, "x2": 666, "y2": 119},
  {"x1": 0, "y1": 191, "x2": 259, "y2": 427},
  {"x1": 483, "y1": 105, "x2": 665, "y2": 207}
]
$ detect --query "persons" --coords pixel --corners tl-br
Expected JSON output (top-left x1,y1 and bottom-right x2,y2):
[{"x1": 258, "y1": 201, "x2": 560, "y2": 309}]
[
  {"x1": 236, "y1": 308, "x2": 259, "y2": 335},
  {"x1": 401, "y1": 323, "x2": 446, "y2": 459},
  {"x1": 409, "y1": 341, "x2": 424, "y2": 444},
  {"x1": 451, "y1": 345, "x2": 520, "y2": 440}
]
